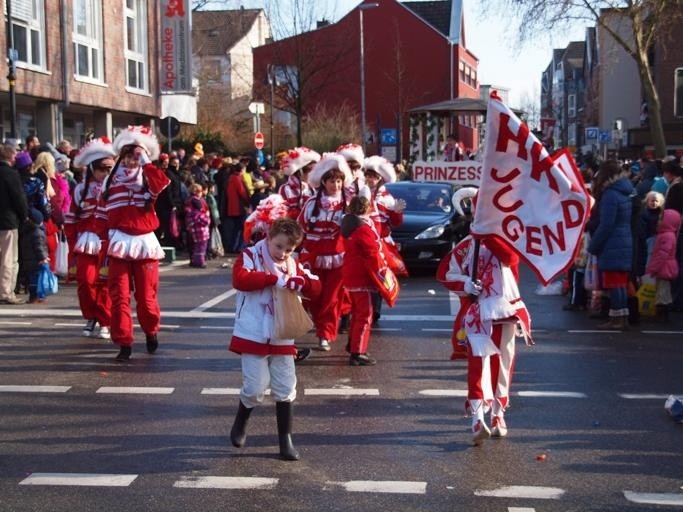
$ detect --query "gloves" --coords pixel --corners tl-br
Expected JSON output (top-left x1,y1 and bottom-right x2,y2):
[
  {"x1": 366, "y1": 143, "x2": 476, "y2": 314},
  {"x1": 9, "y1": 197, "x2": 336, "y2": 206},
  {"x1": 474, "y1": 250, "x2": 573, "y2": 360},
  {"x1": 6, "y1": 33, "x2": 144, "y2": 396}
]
[
  {"x1": 394, "y1": 199, "x2": 406, "y2": 212},
  {"x1": 464, "y1": 276, "x2": 482, "y2": 297},
  {"x1": 287, "y1": 276, "x2": 305, "y2": 291}
]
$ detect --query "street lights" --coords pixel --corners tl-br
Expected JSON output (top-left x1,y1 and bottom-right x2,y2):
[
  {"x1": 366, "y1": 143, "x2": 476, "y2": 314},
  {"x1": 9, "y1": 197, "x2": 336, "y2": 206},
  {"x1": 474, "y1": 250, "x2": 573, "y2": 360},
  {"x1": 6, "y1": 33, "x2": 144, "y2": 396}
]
[
  {"x1": 263, "y1": 64, "x2": 280, "y2": 162},
  {"x1": 358, "y1": 3, "x2": 378, "y2": 158}
]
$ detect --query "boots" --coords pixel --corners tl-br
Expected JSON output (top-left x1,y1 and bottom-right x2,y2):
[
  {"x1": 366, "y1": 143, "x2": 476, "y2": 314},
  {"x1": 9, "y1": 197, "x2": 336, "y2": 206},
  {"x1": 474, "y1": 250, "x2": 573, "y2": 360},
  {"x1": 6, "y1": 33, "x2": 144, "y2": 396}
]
[
  {"x1": 599, "y1": 309, "x2": 630, "y2": 330},
  {"x1": 231, "y1": 401, "x2": 254, "y2": 447},
  {"x1": 491, "y1": 397, "x2": 508, "y2": 437},
  {"x1": 276, "y1": 401, "x2": 299, "y2": 461},
  {"x1": 467, "y1": 399, "x2": 491, "y2": 445}
]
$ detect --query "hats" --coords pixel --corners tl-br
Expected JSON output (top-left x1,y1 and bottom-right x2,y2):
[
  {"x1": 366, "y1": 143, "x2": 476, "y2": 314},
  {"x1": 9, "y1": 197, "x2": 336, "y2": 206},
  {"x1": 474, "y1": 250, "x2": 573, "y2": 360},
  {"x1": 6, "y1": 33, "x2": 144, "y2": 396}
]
[
  {"x1": 73, "y1": 125, "x2": 160, "y2": 168},
  {"x1": 281, "y1": 144, "x2": 396, "y2": 187},
  {"x1": 16, "y1": 151, "x2": 32, "y2": 167}
]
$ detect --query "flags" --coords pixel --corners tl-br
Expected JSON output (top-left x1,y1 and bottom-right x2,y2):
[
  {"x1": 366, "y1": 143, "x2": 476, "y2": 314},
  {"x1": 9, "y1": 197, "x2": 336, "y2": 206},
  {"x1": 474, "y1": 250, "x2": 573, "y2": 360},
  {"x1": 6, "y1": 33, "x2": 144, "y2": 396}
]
[{"x1": 467, "y1": 89, "x2": 592, "y2": 285}]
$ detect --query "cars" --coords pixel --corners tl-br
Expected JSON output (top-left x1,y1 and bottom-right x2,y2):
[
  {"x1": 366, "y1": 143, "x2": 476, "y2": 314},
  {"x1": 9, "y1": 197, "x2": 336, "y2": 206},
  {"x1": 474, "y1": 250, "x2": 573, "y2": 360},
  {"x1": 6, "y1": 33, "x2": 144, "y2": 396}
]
[{"x1": 379, "y1": 181, "x2": 474, "y2": 278}]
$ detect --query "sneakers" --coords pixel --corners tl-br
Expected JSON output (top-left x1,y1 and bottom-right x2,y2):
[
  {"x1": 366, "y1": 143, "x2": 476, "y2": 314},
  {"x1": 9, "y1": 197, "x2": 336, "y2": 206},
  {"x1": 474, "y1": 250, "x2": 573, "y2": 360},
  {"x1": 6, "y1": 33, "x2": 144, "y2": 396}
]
[
  {"x1": 146, "y1": 334, "x2": 157, "y2": 351},
  {"x1": 116, "y1": 346, "x2": 131, "y2": 362},
  {"x1": 345, "y1": 337, "x2": 377, "y2": 366},
  {"x1": 82, "y1": 319, "x2": 95, "y2": 337},
  {"x1": 295, "y1": 348, "x2": 310, "y2": 362},
  {"x1": 319, "y1": 337, "x2": 331, "y2": 351},
  {"x1": 98, "y1": 327, "x2": 110, "y2": 339}
]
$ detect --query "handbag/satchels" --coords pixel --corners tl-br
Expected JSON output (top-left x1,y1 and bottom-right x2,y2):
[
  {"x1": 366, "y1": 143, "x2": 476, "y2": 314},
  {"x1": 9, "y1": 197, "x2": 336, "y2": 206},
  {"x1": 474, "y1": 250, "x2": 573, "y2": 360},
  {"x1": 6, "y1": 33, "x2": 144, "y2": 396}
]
[
  {"x1": 55, "y1": 240, "x2": 69, "y2": 276},
  {"x1": 590, "y1": 291, "x2": 609, "y2": 311},
  {"x1": 273, "y1": 285, "x2": 314, "y2": 338},
  {"x1": 36, "y1": 264, "x2": 58, "y2": 296}
]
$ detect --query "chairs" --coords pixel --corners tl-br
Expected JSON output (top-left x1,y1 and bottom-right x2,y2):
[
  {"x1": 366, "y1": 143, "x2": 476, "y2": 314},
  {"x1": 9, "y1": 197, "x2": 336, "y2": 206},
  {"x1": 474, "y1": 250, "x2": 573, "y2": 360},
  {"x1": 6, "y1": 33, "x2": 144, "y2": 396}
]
[{"x1": 436, "y1": 192, "x2": 450, "y2": 205}]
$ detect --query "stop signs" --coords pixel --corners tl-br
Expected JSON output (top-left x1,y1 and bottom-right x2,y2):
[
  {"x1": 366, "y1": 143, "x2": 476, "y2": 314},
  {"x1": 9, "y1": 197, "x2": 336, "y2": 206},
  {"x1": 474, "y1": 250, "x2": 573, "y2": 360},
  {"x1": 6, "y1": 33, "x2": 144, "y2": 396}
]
[{"x1": 253, "y1": 132, "x2": 264, "y2": 151}]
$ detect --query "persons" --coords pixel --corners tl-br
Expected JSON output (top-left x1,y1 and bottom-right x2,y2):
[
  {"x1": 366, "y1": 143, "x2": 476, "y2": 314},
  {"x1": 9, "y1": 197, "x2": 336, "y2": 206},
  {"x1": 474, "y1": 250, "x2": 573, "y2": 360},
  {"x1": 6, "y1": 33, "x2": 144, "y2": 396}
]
[
  {"x1": 227, "y1": 217, "x2": 323, "y2": 461},
  {"x1": 434, "y1": 189, "x2": 535, "y2": 449},
  {"x1": 555, "y1": 140, "x2": 682, "y2": 330},
  {"x1": 2, "y1": 122, "x2": 408, "y2": 365},
  {"x1": 442, "y1": 133, "x2": 469, "y2": 162},
  {"x1": 429, "y1": 193, "x2": 450, "y2": 212}
]
